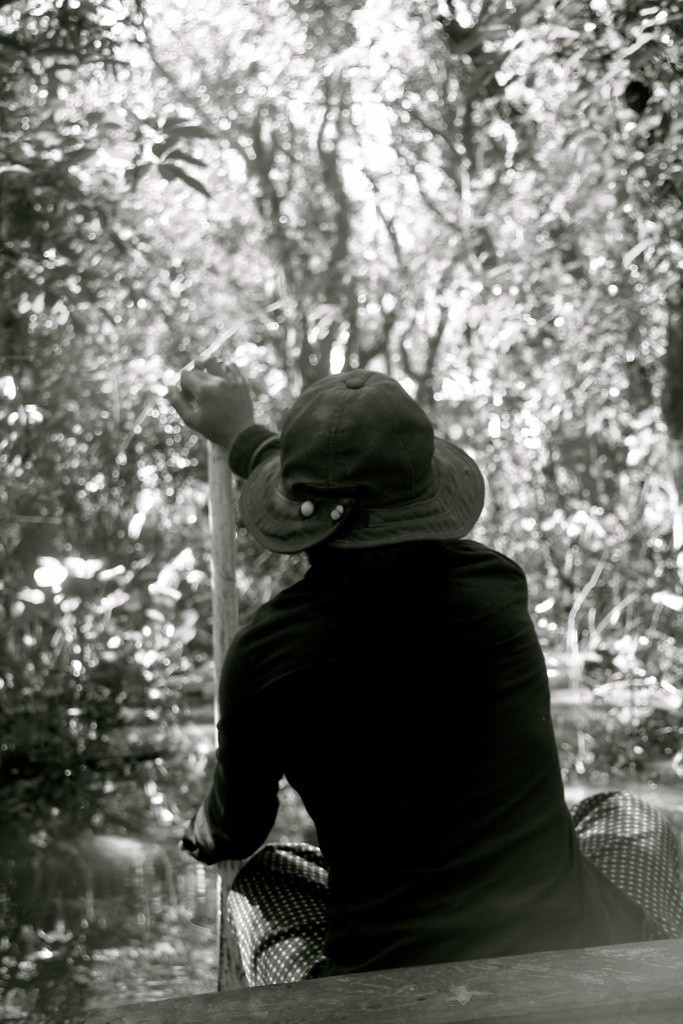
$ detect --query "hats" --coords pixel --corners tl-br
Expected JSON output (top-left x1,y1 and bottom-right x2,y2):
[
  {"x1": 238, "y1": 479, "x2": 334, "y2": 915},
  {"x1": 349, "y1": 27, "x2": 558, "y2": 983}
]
[{"x1": 239, "y1": 370, "x2": 486, "y2": 553}]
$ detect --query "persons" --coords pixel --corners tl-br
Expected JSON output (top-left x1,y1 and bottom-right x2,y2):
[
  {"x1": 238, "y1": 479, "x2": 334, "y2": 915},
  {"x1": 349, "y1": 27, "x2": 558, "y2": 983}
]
[{"x1": 168, "y1": 360, "x2": 681, "y2": 988}]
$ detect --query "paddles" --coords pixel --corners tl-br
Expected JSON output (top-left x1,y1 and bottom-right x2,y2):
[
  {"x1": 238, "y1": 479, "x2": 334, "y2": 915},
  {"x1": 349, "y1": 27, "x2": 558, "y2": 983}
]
[{"x1": 201, "y1": 437, "x2": 243, "y2": 993}]
